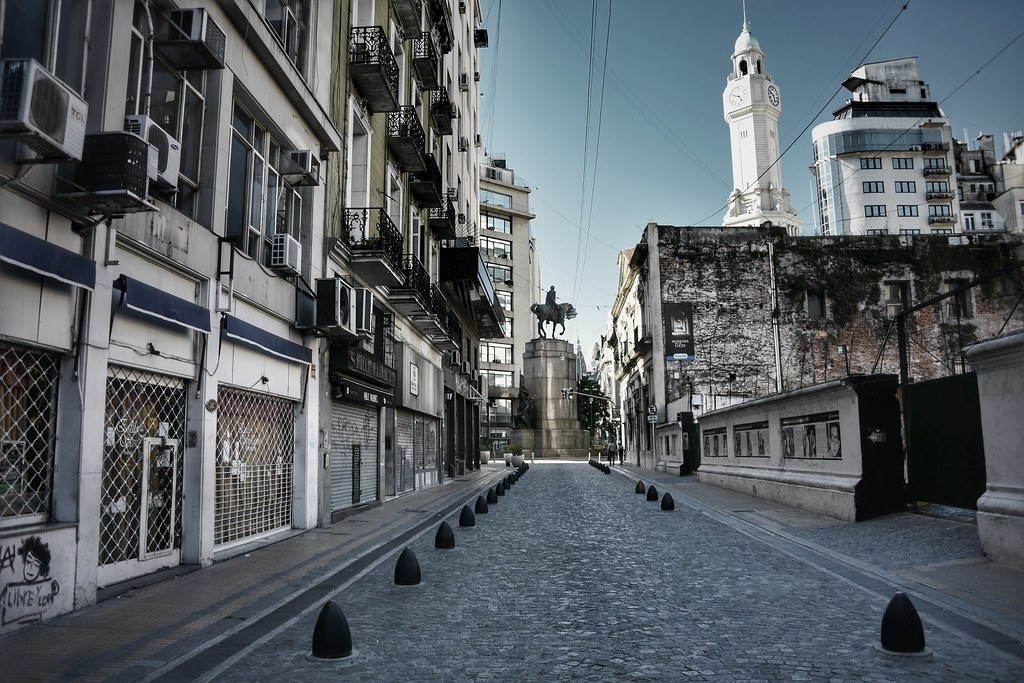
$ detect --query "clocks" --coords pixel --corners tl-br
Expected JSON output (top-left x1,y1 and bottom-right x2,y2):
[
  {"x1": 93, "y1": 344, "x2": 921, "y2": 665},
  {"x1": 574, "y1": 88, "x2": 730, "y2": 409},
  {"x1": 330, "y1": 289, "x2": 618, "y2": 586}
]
[
  {"x1": 728, "y1": 86, "x2": 748, "y2": 106},
  {"x1": 767, "y1": 85, "x2": 779, "y2": 107}
]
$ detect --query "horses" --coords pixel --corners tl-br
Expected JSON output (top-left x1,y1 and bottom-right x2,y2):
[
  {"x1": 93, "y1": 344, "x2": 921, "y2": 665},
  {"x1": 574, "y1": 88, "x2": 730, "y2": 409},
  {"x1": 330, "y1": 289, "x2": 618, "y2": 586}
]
[{"x1": 529, "y1": 303, "x2": 578, "y2": 338}]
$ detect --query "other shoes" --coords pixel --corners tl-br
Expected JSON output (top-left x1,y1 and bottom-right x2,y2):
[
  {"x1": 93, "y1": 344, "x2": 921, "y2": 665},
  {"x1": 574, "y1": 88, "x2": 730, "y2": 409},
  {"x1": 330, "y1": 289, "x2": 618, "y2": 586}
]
[{"x1": 620, "y1": 464, "x2": 623, "y2": 465}]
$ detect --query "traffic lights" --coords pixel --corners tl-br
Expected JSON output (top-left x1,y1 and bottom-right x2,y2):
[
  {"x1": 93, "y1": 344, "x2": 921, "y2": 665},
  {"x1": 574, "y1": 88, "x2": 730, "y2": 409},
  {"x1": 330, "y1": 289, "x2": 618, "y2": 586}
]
[
  {"x1": 562, "y1": 388, "x2": 567, "y2": 400},
  {"x1": 568, "y1": 388, "x2": 573, "y2": 400}
]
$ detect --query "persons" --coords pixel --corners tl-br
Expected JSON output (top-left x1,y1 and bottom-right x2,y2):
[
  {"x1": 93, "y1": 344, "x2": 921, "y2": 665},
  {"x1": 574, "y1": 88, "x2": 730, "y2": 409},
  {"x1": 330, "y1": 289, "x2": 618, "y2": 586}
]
[
  {"x1": 616, "y1": 443, "x2": 625, "y2": 465},
  {"x1": 517, "y1": 383, "x2": 529, "y2": 414},
  {"x1": 546, "y1": 286, "x2": 560, "y2": 310},
  {"x1": 607, "y1": 440, "x2": 616, "y2": 467}
]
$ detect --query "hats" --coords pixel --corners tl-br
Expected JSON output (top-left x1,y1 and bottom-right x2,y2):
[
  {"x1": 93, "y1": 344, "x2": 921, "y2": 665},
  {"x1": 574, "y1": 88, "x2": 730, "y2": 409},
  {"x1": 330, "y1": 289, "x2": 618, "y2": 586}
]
[{"x1": 609, "y1": 440, "x2": 613, "y2": 443}]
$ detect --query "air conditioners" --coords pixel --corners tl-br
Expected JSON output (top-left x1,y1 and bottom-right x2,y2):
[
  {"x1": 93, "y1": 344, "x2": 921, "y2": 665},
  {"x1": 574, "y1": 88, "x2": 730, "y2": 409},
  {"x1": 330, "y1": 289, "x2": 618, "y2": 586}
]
[
  {"x1": 448, "y1": 187, "x2": 458, "y2": 200},
  {"x1": 124, "y1": 115, "x2": 182, "y2": 190},
  {"x1": 459, "y1": 214, "x2": 466, "y2": 223},
  {"x1": 0, "y1": 55, "x2": 88, "y2": 163},
  {"x1": 460, "y1": 72, "x2": 470, "y2": 91},
  {"x1": 475, "y1": 134, "x2": 482, "y2": 146},
  {"x1": 475, "y1": 72, "x2": 480, "y2": 80},
  {"x1": 287, "y1": 150, "x2": 320, "y2": 185},
  {"x1": 459, "y1": 1, "x2": 466, "y2": 13},
  {"x1": 450, "y1": 101, "x2": 457, "y2": 117},
  {"x1": 460, "y1": 136, "x2": 469, "y2": 150},
  {"x1": 913, "y1": 141, "x2": 956, "y2": 223},
  {"x1": 467, "y1": 235, "x2": 475, "y2": 244},
  {"x1": 451, "y1": 351, "x2": 479, "y2": 382},
  {"x1": 314, "y1": 278, "x2": 356, "y2": 334},
  {"x1": 178, "y1": 7, "x2": 228, "y2": 63},
  {"x1": 353, "y1": 287, "x2": 374, "y2": 332},
  {"x1": 271, "y1": 234, "x2": 303, "y2": 276}
]
